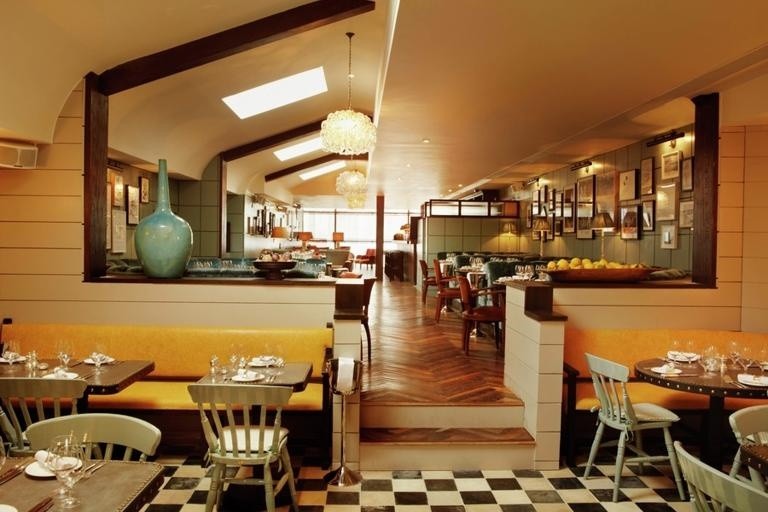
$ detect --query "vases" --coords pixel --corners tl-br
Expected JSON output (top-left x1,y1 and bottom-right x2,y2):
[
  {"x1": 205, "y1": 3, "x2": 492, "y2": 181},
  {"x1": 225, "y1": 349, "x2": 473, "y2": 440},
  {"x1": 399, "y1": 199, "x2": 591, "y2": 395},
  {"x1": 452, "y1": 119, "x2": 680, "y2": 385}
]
[{"x1": 134, "y1": 158, "x2": 194, "y2": 278}]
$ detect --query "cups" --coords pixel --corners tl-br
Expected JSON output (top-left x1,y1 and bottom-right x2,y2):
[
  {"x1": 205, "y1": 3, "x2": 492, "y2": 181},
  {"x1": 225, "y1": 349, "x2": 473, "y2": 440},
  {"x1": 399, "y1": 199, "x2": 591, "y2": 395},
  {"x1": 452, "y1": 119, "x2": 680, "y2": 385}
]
[
  {"x1": 209, "y1": 342, "x2": 251, "y2": 374},
  {"x1": 447, "y1": 253, "x2": 456, "y2": 260},
  {"x1": 192, "y1": 260, "x2": 326, "y2": 275},
  {"x1": 0, "y1": 434, "x2": 7, "y2": 469},
  {"x1": 1, "y1": 338, "x2": 107, "y2": 374}
]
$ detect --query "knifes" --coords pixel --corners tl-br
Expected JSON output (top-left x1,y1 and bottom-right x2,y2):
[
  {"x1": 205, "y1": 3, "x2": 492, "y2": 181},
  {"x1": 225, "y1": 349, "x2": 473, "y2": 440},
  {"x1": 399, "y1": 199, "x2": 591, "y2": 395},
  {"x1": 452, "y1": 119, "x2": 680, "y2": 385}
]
[
  {"x1": 0, "y1": 456, "x2": 37, "y2": 484},
  {"x1": 74, "y1": 458, "x2": 107, "y2": 483},
  {"x1": 110, "y1": 360, "x2": 125, "y2": 367},
  {"x1": 0, "y1": 455, "x2": 34, "y2": 480},
  {"x1": 78, "y1": 370, "x2": 97, "y2": 380},
  {"x1": 661, "y1": 373, "x2": 699, "y2": 377},
  {"x1": 267, "y1": 374, "x2": 276, "y2": 384},
  {"x1": 37, "y1": 500, "x2": 54, "y2": 512},
  {"x1": 27, "y1": 495, "x2": 54, "y2": 512}
]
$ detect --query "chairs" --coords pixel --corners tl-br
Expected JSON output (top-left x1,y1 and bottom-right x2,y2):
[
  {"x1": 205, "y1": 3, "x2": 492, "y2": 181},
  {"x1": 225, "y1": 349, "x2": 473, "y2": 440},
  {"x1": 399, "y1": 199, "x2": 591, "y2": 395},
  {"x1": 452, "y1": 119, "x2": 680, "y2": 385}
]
[
  {"x1": 186, "y1": 384, "x2": 297, "y2": 512},
  {"x1": 119, "y1": 246, "x2": 377, "y2": 364},
  {"x1": 674, "y1": 405, "x2": 768, "y2": 512},
  {"x1": 419, "y1": 252, "x2": 595, "y2": 354},
  {"x1": 25, "y1": 413, "x2": 162, "y2": 460},
  {"x1": 0, "y1": 376, "x2": 102, "y2": 461},
  {"x1": 582, "y1": 352, "x2": 686, "y2": 503}
]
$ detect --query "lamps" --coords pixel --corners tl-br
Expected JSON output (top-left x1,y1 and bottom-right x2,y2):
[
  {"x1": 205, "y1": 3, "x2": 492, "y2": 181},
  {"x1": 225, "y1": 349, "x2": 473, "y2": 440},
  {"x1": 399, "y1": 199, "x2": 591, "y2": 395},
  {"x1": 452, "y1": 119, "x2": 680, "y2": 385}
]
[
  {"x1": 272, "y1": 227, "x2": 345, "y2": 248},
  {"x1": 319, "y1": 31, "x2": 375, "y2": 209}
]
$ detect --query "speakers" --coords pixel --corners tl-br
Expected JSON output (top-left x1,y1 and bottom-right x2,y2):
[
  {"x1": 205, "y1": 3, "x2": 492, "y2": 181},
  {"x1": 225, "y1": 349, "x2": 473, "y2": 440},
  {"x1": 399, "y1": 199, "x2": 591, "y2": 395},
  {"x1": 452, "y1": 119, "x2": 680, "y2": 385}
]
[{"x1": 0, "y1": 141, "x2": 39, "y2": 169}]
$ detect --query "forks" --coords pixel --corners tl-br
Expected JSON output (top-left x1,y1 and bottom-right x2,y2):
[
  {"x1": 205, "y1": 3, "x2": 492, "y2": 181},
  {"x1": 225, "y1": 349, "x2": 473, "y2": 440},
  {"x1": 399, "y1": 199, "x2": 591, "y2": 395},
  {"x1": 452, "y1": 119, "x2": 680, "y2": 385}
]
[{"x1": 721, "y1": 374, "x2": 746, "y2": 389}]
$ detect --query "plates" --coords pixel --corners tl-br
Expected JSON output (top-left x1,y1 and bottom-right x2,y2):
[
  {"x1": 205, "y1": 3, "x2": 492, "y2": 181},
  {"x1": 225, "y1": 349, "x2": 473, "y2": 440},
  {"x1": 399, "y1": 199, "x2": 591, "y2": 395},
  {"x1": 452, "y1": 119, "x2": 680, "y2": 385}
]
[
  {"x1": 232, "y1": 374, "x2": 265, "y2": 381},
  {"x1": 83, "y1": 357, "x2": 114, "y2": 365},
  {"x1": 737, "y1": 376, "x2": 768, "y2": 386},
  {"x1": 42, "y1": 372, "x2": 78, "y2": 380},
  {"x1": 651, "y1": 367, "x2": 682, "y2": 374},
  {"x1": 0, "y1": 503, "x2": 18, "y2": 512},
  {"x1": 25, "y1": 457, "x2": 82, "y2": 477},
  {"x1": 248, "y1": 361, "x2": 272, "y2": 367}
]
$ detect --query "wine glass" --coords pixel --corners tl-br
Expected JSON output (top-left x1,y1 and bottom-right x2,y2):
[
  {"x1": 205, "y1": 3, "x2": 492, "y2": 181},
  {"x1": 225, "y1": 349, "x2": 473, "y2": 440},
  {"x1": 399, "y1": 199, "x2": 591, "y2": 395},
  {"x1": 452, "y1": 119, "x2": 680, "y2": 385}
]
[
  {"x1": 469, "y1": 256, "x2": 483, "y2": 270},
  {"x1": 514, "y1": 263, "x2": 545, "y2": 281},
  {"x1": 46, "y1": 433, "x2": 85, "y2": 510},
  {"x1": 668, "y1": 336, "x2": 768, "y2": 376}
]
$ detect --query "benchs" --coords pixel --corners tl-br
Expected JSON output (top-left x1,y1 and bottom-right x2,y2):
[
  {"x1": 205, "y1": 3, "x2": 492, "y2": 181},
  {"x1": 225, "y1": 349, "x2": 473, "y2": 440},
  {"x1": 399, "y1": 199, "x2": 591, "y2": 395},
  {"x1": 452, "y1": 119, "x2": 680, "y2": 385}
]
[
  {"x1": 562, "y1": 330, "x2": 768, "y2": 457},
  {"x1": 0, "y1": 322, "x2": 333, "y2": 455}
]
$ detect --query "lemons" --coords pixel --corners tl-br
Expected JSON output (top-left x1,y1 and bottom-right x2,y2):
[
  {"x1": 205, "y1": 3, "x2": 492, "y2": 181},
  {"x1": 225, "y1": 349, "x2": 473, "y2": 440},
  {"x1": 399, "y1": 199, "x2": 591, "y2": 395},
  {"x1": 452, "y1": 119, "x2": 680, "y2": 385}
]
[{"x1": 547, "y1": 258, "x2": 628, "y2": 269}]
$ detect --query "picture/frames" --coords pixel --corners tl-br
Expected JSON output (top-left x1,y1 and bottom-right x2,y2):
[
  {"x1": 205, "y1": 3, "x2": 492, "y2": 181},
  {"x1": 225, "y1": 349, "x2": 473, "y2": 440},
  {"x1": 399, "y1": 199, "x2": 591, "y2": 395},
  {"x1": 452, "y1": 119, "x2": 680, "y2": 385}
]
[
  {"x1": 249, "y1": 206, "x2": 280, "y2": 238},
  {"x1": 526, "y1": 151, "x2": 694, "y2": 249},
  {"x1": 106, "y1": 167, "x2": 150, "y2": 255}
]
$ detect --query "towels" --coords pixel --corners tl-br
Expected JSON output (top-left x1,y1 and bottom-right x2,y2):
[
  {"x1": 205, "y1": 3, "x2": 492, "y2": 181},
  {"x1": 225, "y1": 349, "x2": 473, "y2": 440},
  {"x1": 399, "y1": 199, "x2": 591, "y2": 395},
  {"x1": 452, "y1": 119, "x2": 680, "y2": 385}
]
[
  {"x1": 657, "y1": 351, "x2": 768, "y2": 385},
  {"x1": 2, "y1": 350, "x2": 106, "y2": 378},
  {"x1": 237, "y1": 369, "x2": 259, "y2": 378},
  {"x1": 336, "y1": 357, "x2": 355, "y2": 391},
  {"x1": 35, "y1": 449, "x2": 73, "y2": 471}
]
